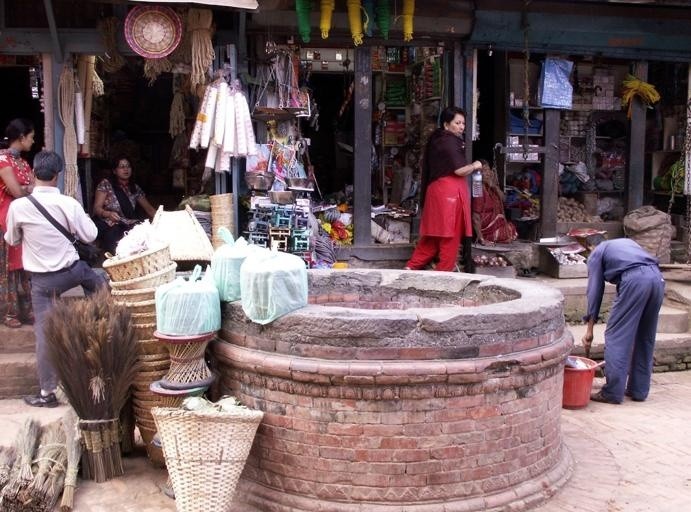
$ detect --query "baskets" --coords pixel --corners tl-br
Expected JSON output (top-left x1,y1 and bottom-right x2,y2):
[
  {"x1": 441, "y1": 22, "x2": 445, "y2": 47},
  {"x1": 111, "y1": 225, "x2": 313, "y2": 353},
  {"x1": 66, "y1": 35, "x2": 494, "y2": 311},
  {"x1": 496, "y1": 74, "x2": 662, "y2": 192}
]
[
  {"x1": 125, "y1": 4, "x2": 182, "y2": 59},
  {"x1": 102, "y1": 245, "x2": 177, "y2": 468}
]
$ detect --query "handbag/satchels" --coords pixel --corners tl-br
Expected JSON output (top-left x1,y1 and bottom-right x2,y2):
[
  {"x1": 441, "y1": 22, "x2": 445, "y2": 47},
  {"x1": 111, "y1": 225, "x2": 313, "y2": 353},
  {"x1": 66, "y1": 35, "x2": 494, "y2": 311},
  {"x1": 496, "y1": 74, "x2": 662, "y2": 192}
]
[{"x1": 73, "y1": 241, "x2": 101, "y2": 268}]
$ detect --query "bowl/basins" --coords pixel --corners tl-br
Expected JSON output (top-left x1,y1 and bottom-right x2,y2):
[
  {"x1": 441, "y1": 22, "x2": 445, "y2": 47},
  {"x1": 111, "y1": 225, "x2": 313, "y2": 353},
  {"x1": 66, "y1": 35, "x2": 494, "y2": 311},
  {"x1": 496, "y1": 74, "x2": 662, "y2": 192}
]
[
  {"x1": 267, "y1": 190, "x2": 299, "y2": 205},
  {"x1": 284, "y1": 177, "x2": 314, "y2": 188},
  {"x1": 244, "y1": 172, "x2": 275, "y2": 191}
]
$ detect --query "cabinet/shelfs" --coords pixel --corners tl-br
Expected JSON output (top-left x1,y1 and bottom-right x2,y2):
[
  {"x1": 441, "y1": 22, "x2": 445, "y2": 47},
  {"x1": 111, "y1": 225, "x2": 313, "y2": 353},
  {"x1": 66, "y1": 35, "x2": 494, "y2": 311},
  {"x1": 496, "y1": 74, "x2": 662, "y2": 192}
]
[
  {"x1": 371, "y1": 46, "x2": 442, "y2": 207},
  {"x1": 496, "y1": 48, "x2": 628, "y2": 196},
  {"x1": 645, "y1": 105, "x2": 684, "y2": 198}
]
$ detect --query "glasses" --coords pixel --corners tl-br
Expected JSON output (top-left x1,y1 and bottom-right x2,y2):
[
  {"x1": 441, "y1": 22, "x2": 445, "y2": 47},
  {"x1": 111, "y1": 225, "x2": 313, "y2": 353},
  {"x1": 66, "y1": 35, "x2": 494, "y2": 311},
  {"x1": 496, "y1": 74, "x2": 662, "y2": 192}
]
[{"x1": 117, "y1": 163, "x2": 131, "y2": 168}]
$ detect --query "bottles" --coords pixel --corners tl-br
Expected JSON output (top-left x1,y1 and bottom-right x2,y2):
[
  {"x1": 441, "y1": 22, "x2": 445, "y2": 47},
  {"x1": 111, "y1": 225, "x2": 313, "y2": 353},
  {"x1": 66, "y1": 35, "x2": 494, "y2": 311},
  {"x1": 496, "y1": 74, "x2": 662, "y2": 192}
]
[
  {"x1": 472, "y1": 170, "x2": 483, "y2": 197},
  {"x1": 669, "y1": 136, "x2": 675, "y2": 151}
]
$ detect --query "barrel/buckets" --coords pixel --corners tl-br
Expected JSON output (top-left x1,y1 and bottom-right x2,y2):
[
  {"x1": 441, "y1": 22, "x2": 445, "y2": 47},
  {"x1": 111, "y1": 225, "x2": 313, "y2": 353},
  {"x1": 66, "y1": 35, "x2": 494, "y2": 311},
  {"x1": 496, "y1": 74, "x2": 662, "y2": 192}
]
[{"x1": 562, "y1": 354, "x2": 598, "y2": 408}]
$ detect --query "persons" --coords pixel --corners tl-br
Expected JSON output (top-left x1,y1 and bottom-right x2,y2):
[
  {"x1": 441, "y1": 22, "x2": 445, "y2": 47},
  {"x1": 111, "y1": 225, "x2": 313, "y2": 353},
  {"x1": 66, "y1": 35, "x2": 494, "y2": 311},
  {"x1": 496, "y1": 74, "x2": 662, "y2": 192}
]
[
  {"x1": 3, "y1": 150, "x2": 102, "y2": 409},
  {"x1": 424, "y1": 105, "x2": 483, "y2": 274},
  {"x1": 580, "y1": 231, "x2": 665, "y2": 405},
  {"x1": 1, "y1": 118, "x2": 39, "y2": 327},
  {"x1": 90, "y1": 155, "x2": 159, "y2": 255}
]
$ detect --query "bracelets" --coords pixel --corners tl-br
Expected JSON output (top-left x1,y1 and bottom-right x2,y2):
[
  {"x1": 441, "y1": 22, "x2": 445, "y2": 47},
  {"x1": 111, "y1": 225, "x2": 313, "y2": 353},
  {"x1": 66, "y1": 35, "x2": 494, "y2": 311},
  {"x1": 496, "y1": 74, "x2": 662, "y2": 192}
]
[{"x1": 471, "y1": 163, "x2": 476, "y2": 170}]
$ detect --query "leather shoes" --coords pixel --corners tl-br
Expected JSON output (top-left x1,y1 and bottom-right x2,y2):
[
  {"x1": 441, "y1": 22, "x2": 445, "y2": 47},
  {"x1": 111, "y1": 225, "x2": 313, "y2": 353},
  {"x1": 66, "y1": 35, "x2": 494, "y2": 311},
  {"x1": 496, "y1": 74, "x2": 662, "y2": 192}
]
[
  {"x1": 23, "y1": 390, "x2": 59, "y2": 409},
  {"x1": 590, "y1": 387, "x2": 622, "y2": 405}
]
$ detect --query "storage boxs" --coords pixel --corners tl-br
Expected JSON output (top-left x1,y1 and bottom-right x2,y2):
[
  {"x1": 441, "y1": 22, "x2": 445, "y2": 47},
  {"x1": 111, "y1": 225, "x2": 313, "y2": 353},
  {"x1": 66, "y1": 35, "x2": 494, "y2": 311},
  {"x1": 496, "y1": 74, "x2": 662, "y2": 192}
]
[
  {"x1": 538, "y1": 247, "x2": 588, "y2": 279},
  {"x1": 473, "y1": 266, "x2": 517, "y2": 279}
]
